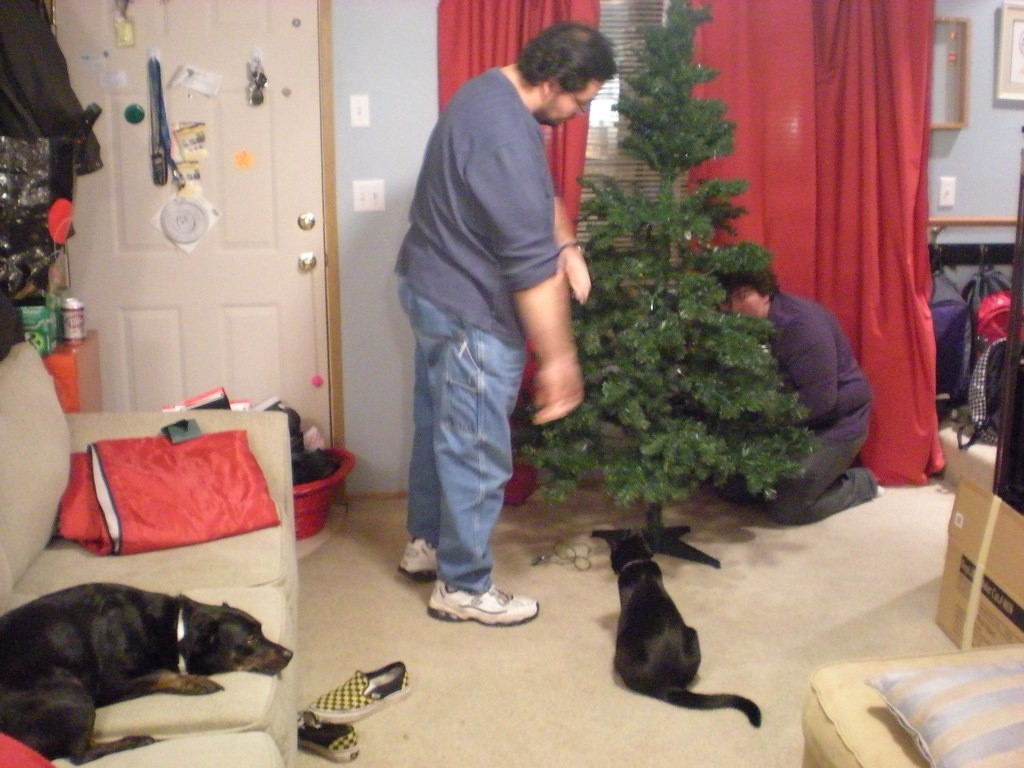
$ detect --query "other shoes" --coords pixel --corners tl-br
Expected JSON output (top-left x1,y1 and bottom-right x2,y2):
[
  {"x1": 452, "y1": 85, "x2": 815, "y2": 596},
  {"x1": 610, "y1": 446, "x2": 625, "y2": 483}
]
[
  {"x1": 296, "y1": 710, "x2": 360, "y2": 762},
  {"x1": 306, "y1": 661, "x2": 410, "y2": 724}
]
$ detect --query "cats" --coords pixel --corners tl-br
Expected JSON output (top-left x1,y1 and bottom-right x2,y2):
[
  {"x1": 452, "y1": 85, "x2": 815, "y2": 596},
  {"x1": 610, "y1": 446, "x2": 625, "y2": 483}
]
[{"x1": 608, "y1": 531, "x2": 761, "y2": 729}]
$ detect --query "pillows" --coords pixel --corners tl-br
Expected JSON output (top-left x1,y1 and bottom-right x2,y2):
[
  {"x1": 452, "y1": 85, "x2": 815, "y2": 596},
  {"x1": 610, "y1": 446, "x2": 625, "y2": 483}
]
[{"x1": 868, "y1": 661, "x2": 1024, "y2": 768}]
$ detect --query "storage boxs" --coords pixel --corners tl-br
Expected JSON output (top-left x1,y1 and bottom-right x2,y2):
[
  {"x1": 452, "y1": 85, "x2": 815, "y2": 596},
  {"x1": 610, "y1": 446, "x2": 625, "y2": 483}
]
[{"x1": 937, "y1": 479, "x2": 1024, "y2": 651}]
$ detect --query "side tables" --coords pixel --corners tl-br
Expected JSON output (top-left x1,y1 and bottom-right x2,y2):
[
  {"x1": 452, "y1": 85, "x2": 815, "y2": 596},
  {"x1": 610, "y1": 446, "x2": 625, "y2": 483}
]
[{"x1": 43, "y1": 327, "x2": 105, "y2": 413}]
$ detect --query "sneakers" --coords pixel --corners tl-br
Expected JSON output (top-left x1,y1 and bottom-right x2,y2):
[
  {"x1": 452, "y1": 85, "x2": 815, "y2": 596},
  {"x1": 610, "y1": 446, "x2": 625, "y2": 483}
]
[
  {"x1": 396, "y1": 537, "x2": 442, "y2": 582},
  {"x1": 427, "y1": 578, "x2": 539, "y2": 626}
]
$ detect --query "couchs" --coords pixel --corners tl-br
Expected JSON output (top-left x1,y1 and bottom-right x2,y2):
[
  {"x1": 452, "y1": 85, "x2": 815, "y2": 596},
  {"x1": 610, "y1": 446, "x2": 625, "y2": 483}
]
[
  {"x1": 801, "y1": 647, "x2": 1024, "y2": 768},
  {"x1": 0, "y1": 350, "x2": 299, "y2": 768}
]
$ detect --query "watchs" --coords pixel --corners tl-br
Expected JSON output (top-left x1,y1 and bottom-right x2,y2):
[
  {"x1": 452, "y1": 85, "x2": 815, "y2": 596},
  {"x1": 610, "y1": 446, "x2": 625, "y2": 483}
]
[{"x1": 556, "y1": 243, "x2": 584, "y2": 257}]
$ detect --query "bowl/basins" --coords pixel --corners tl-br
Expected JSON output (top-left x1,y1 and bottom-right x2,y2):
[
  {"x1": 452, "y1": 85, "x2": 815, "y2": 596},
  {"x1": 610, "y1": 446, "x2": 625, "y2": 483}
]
[{"x1": 295, "y1": 448, "x2": 357, "y2": 539}]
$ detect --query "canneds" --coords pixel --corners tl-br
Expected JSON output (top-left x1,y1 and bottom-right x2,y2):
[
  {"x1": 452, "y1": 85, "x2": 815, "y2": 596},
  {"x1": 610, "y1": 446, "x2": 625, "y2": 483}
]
[{"x1": 61, "y1": 297, "x2": 85, "y2": 340}]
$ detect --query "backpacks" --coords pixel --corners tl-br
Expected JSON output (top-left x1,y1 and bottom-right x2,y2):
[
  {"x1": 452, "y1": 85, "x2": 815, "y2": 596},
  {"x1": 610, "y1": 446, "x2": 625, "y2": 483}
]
[{"x1": 932, "y1": 248, "x2": 1024, "y2": 447}]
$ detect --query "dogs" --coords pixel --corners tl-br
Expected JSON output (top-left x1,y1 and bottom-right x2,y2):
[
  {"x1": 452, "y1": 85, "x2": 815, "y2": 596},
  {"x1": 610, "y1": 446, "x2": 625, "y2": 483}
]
[{"x1": 0, "y1": 583, "x2": 291, "y2": 764}]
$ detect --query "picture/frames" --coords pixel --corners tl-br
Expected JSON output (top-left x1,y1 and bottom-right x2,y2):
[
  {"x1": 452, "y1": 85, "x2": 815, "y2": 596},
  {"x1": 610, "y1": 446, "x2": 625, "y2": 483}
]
[{"x1": 994, "y1": 3, "x2": 1024, "y2": 101}]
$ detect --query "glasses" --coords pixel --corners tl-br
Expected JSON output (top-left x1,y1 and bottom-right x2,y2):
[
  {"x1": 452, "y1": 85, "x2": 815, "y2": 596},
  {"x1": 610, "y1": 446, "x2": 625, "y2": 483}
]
[{"x1": 565, "y1": 80, "x2": 591, "y2": 115}]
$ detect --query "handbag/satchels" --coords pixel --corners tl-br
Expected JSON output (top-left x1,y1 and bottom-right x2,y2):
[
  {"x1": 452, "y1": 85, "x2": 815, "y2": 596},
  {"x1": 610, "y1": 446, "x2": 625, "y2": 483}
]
[{"x1": 0, "y1": 80, "x2": 76, "y2": 301}]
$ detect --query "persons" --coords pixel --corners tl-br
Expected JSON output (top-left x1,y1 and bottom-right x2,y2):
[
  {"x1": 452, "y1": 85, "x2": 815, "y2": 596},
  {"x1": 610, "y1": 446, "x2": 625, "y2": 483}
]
[
  {"x1": 392, "y1": 22, "x2": 617, "y2": 627},
  {"x1": 714, "y1": 246, "x2": 883, "y2": 528}
]
